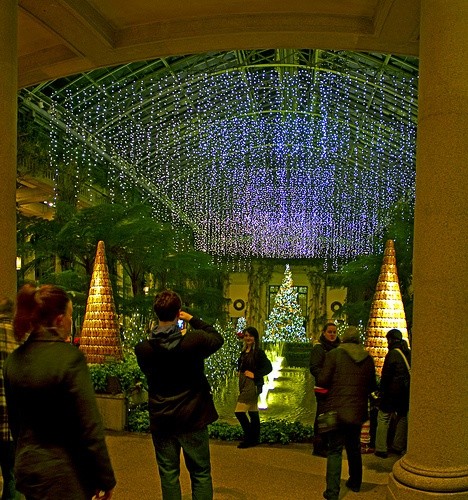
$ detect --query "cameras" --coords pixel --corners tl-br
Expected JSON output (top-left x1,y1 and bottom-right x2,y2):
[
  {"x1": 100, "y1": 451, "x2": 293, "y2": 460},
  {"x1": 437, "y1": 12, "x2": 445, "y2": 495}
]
[{"x1": 177, "y1": 319, "x2": 187, "y2": 329}]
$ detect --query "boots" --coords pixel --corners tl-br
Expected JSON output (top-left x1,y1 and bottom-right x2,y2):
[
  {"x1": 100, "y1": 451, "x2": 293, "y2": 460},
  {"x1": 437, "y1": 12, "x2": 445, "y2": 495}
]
[
  {"x1": 237, "y1": 411, "x2": 260, "y2": 449},
  {"x1": 312, "y1": 438, "x2": 328, "y2": 457},
  {"x1": 234, "y1": 412, "x2": 251, "y2": 444}
]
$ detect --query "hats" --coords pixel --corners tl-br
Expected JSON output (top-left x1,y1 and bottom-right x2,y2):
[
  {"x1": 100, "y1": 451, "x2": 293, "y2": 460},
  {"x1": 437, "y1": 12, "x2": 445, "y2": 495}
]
[
  {"x1": 386, "y1": 329, "x2": 402, "y2": 340},
  {"x1": 401, "y1": 339, "x2": 408, "y2": 351},
  {"x1": 343, "y1": 327, "x2": 360, "y2": 342}
]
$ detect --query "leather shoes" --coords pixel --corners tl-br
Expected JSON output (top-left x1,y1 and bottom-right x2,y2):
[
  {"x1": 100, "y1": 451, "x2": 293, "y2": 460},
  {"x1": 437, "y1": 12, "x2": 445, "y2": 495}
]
[
  {"x1": 375, "y1": 451, "x2": 388, "y2": 459},
  {"x1": 346, "y1": 480, "x2": 361, "y2": 492},
  {"x1": 323, "y1": 490, "x2": 338, "y2": 500},
  {"x1": 387, "y1": 447, "x2": 402, "y2": 456}
]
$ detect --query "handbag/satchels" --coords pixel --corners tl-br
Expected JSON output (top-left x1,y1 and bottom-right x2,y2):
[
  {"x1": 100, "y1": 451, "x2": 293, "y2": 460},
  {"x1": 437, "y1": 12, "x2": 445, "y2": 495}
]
[{"x1": 13, "y1": 425, "x2": 87, "y2": 500}]
